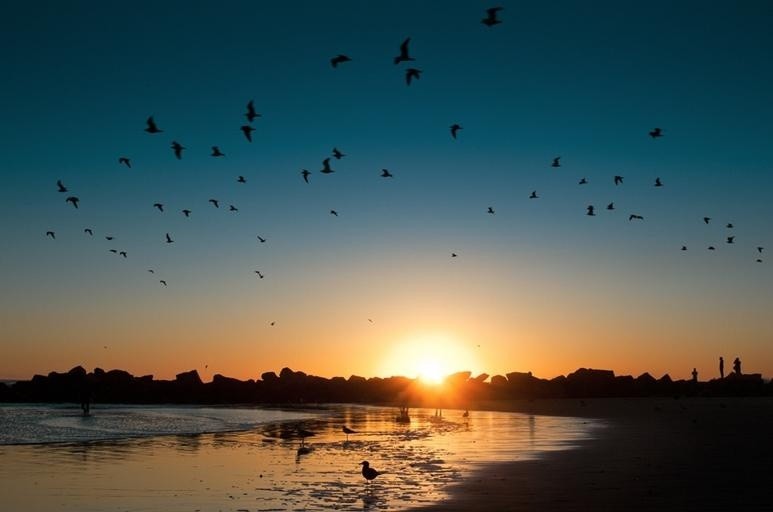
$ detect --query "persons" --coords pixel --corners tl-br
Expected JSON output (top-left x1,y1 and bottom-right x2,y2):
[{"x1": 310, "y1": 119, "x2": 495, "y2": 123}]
[
  {"x1": 719, "y1": 356, "x2": 724, "y2": 378},
  {"x1": 732, "y1": 357, "x2": 741, "y2": 374},
  {"x1": 691, "y1": 367, "x2": 698, "y2": 380}
]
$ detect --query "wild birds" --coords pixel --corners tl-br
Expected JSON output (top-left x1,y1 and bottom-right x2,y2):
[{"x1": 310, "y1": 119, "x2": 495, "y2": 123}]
[
  {"x1": 293, "y1": 427, "x2": 315, "y2": 442},
  {"x1": 358, "y1": 460, "x2": 380, "y2": 483},
  {"x1": 146, "y1": 174, "x2": 263, "y2": 285},
  {"x1": 480, "y1": 5, "x2": 504, "y2": 26},
  {"x1": 341, "y1": 424, "x2": 353, "y2": 439},
  {"x1": 65, "y1": 197, "x2": 81, "y2": 209},
  {"x1": 170, "y1": 141, "x2": 185, "y2": 159},
  {"x1": 448, "y1": 121, "x2": 462, "y2": 140},
  {"x1": 117, "y1": 158, "x2": 131, "y2": 168},
  {"x1": 330, "y1": 208, "x2": 340, "y2": 219},
  {"x1": 240, "y1": 125, "x2": 256, "y2": 143},
  {"x1": 392, "y1": 38, "x2": 426, "y2": 89},
  {"x1": 209, "y1": 146, "x2": 225, "y2": 157},
  {"x1": 244, "y1": 99, "x2": 261, "y2": 122},
  {"x1": 379, "y1": 169, "x2": 393, "y2": 178},
  {"x1": 328, "y1": 54, "x2": 354, "y2": 67},
  {"x1": 300, "y1": 148, "x2": 345, "y2": 184},
  {"x1": 46, "y1": 231, "x2": 55, "y2": 239},
  {"x1": 57, "y1": 180, "x2": 71, "y2": 193},
  {"x1": 485, "y1": 126, "x2": 764, "y2": 264},
  {"x1": 143, "y1": 116, "x2": 164, "y2": 133},
  {"x1": 84, "y1": 229, "x2": 127, "y2": 259}
]
